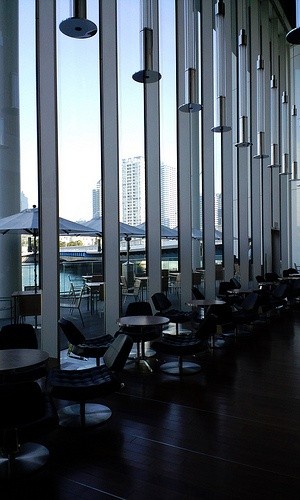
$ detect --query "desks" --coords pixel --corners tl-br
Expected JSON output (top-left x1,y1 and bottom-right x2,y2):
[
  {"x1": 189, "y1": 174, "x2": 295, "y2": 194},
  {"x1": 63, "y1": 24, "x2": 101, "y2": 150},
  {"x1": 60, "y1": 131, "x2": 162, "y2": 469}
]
[
  {"x1": 259, "y1": 281, "x2": 281, "y2": 318},
  {"x1": 134, "y1": 277, "x2": 149, "y2": 302},
  {"x1": 87, "y1": 282, "x2": 123, "y2": 314},
  {"x1": 227, "y1": 288, "x2": 261, "y2": 329},
  {"x1": 58, "y1": 348, "x2": 113, "y2": 427},
  {"x1": 0, "y1": 348, "x2": 50, "y2": 479},
  {"x1": 187, "y1": 299, "x2": 228, "y2": 352},
  {"x1": 278, "y1": 277, "x2": 300, "y2": 309},
  {"x1": 11, "y1": 290, "x2": 41, "y2": 325},
  {"x1": 157, "y1": 328, "x2": 200, "y2": 373},
  {"x1": 116, "y1": 315, "x2": 171, "y2": 360}
]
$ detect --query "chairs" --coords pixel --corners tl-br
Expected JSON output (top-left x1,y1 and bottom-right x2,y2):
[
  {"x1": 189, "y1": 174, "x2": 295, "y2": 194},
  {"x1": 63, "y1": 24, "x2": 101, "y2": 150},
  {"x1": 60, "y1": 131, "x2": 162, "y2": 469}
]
[{"x1": 0, "y1": 264, "x2": 300, "y2": 436}]
